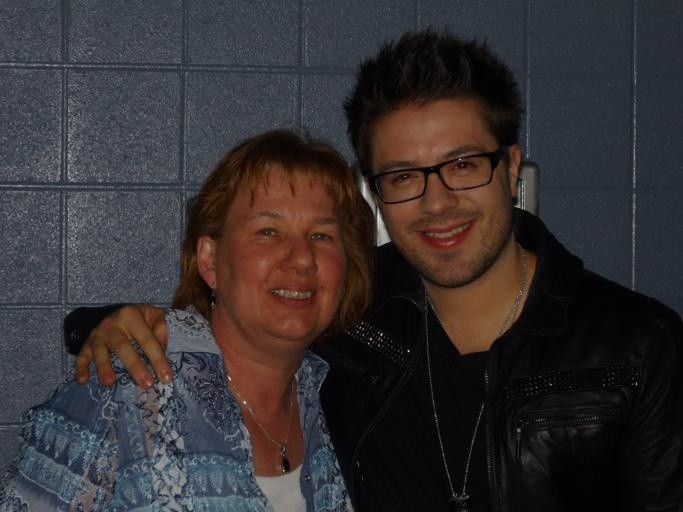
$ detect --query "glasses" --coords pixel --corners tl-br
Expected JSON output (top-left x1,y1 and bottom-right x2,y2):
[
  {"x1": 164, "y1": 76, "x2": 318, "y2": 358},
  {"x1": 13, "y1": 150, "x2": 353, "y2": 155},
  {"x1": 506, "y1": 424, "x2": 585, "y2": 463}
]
[{"x1": 369, "y1": 147, "x2": 504, "y2": 204}]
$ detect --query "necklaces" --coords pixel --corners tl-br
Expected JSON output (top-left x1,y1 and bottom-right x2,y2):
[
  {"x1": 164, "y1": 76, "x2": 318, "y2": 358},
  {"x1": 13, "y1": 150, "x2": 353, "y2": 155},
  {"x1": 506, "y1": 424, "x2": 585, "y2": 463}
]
[
  {"x1": 225, "y1": 369, "x2": 299, "y2": 474},
  {"x1": 419, "y1": 238, "x2": 529, "y2": 511}
]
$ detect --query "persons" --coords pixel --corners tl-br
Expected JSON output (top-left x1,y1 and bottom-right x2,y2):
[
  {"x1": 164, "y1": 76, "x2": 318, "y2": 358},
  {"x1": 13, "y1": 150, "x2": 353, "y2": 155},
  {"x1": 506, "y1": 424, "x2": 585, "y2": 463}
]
[
  {"x1": 1, "y1": 125, "x2": 380, "y2": 512},
  {"x1": 57, "y1": 27, "x2": 681, "y2": 511}
]
